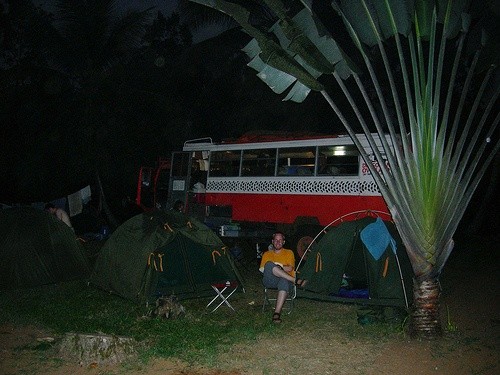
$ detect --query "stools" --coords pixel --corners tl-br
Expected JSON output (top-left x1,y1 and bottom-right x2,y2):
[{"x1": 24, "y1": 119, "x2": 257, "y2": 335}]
[{"x1": 205, "y1": 281, "x2": 239, "y2": 312}]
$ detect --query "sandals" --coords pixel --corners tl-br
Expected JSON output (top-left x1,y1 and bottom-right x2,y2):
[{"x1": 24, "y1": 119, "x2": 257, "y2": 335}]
[
  {"x1": 294, "y1": 278, "x2": 308, "y2": 288},
  {"x1": 272, "y1": 312, "x2": 282, "y2": 323}
]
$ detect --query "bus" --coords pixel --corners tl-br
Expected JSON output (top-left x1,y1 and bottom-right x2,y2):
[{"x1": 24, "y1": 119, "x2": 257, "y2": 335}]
[{"x1": 136, "y1": 129, "x2": 410, "y2": 267}]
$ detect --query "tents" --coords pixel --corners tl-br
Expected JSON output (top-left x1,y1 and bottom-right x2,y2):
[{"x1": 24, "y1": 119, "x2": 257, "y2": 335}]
[
  {"x1": 0, "y1": 207, "x2": 91, "y2": 288},
  {"x1": 298, "y1": 210, "x2": 413, "y2": 309},
  {"x1": 90, "y1": 210, "x2": 246, "y2": 304}
]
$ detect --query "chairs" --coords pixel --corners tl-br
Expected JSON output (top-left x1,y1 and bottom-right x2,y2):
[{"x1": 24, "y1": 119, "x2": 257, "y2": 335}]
[{"x1": 262, "y1": 271, "x2": 297, "y2": 315}]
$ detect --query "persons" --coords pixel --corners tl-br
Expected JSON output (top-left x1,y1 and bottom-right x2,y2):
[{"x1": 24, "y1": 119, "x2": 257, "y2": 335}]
[
  {"x1": 44, "y1": 203, "x2": 75, "y2": 235},
  {"x1": 173, "y1": 200, "x2": 185, "y2": 214},
  {"x1": 190, "y1": 152, "x2": 209, "y2": 187},
  {"x1": 260, "y1": 231, "x2": 297, "y2": 325}
]
